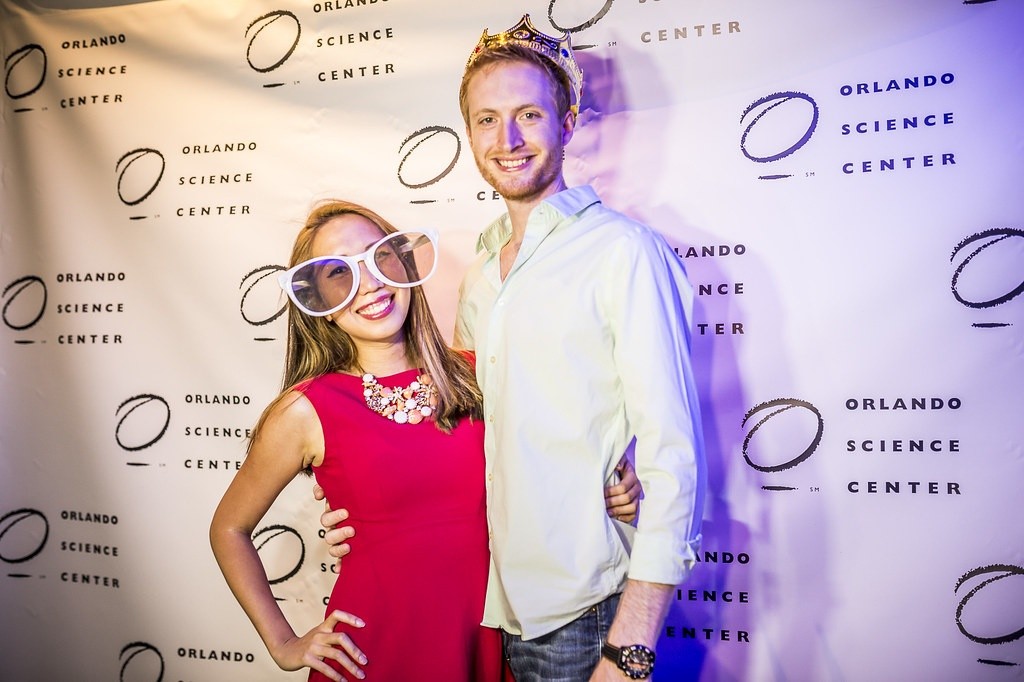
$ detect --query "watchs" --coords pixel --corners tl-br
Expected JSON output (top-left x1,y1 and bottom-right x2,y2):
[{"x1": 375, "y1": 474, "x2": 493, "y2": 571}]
[{"x1": 602, "y1": 644, "x2": 655, "y2": 679}]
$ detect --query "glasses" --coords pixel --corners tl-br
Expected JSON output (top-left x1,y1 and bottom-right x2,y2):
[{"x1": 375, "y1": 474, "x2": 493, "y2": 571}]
[{"x1": 275, "y1": 227, "x2": 440, "y2": 317}]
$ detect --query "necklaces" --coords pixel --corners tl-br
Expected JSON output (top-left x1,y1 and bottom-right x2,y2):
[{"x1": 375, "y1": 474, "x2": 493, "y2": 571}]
[{"x1": 360, "y1": 372, "x2": 438, "y2": 424}]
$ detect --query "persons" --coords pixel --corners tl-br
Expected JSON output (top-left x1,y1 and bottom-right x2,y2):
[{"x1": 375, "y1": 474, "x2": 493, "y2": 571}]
[
  {"x1": 313, "y1": 14, "x2": 705, "y2": 682},
  {"x1": 210, "y1": 200, "x2": 641, "y2": 682}
]
